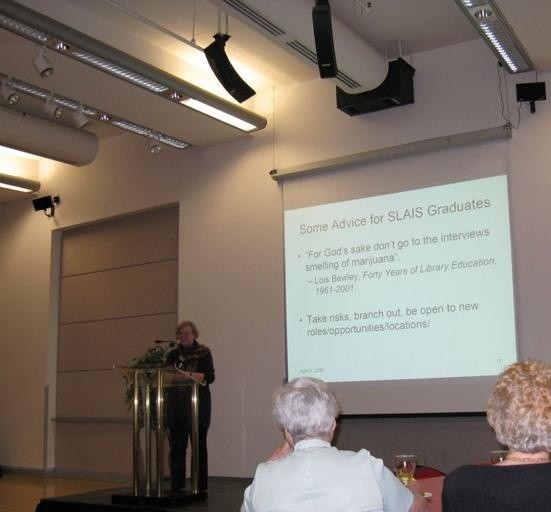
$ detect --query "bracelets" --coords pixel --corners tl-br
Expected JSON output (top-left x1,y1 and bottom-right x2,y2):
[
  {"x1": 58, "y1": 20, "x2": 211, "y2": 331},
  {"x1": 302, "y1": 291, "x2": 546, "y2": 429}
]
[{"x1": 190, "y1": 371, "x2": 192, "y2": 376}]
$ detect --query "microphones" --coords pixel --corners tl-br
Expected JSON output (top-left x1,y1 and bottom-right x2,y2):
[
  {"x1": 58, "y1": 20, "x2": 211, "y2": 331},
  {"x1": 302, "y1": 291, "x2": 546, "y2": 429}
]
[{"x1": 159, "y1": 342, "x2": 178, "y2": 360}]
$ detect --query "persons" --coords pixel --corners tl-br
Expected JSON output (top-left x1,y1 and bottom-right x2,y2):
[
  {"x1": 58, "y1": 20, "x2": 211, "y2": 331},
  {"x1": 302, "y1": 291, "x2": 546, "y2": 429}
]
[
  {"x1": 441, "y1": 358, "x2": 550, "y2": 512},
  {"x1": 162, "y1": 319, "x2": 216, "y2": 492},
  {"x1": 238, "y1": 375, "x2": 431, "y2": 512}
]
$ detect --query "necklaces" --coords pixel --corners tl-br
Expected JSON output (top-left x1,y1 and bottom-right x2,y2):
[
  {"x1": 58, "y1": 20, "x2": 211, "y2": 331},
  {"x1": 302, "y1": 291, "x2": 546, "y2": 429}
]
[{"x1": 503, "y1": 454, "x2": 550, "y2": 463}]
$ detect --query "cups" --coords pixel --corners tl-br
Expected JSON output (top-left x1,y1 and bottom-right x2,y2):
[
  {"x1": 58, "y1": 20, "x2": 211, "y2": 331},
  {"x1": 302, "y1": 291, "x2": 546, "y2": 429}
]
[
  {"x1": 396, "y1": 455, "x2": 416, "y2": 486},
  {"x1": 492, "y1": 451, "x2": 507, "y2": 464}
]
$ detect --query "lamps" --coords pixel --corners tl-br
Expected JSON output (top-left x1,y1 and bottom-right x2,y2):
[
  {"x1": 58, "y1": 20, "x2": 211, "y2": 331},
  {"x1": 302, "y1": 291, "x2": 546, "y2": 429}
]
[
  {"x1": 145, "y1": 131, "x2": 162, "y2": 154},
  {"x1": 0, "y1": 45, "x2": 91, "y2": 129}
]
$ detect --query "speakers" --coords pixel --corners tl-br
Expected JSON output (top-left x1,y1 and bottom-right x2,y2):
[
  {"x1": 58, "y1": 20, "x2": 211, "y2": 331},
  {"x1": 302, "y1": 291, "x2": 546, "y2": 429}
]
[
  {"x1": 201, "y1": 38, "x2": 256, "y2": 103},
  {"x1": 516, "y1": 82, "x2": 548, "y2": 110},
  {"x1": 311, "y1": 3, "x2": 338, "y2": 78},
  {"x1": 32, "y1": 194, "x2": 55, "y2": 217}
]
